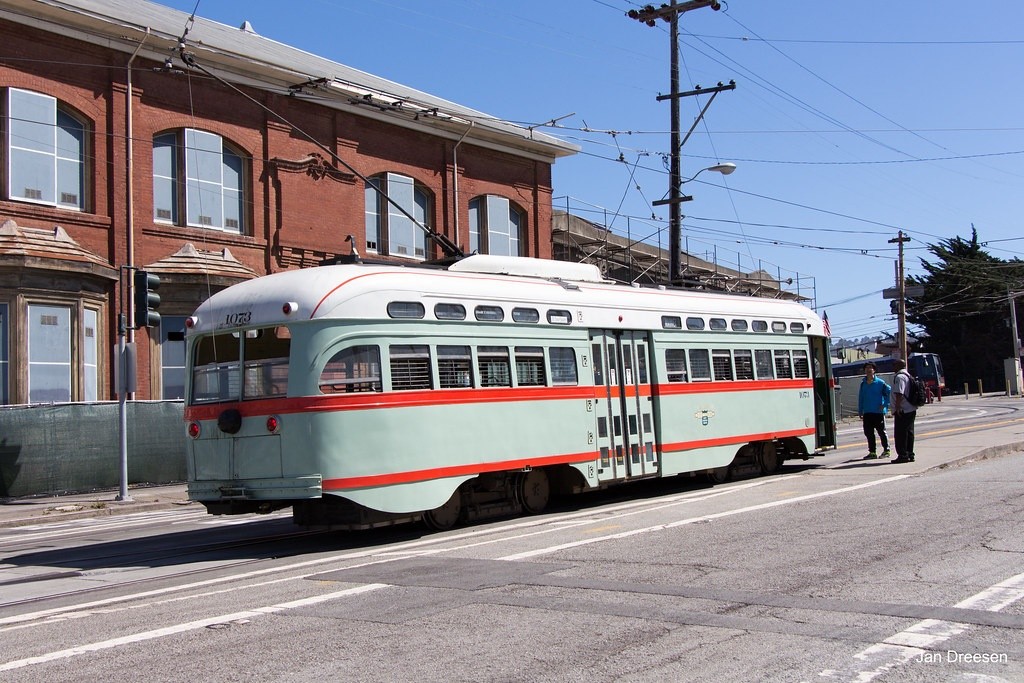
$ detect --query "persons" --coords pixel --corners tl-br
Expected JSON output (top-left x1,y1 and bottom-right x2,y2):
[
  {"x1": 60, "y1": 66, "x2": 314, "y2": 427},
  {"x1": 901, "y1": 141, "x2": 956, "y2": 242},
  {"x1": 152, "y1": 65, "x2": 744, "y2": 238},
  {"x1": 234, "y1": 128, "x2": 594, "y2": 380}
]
[
  {"x1": 891, "y1": 359, "x2": 918, "y2": 463},
  {"x1": 857, "y1": 360, "x2": 892, "y2": 460}
]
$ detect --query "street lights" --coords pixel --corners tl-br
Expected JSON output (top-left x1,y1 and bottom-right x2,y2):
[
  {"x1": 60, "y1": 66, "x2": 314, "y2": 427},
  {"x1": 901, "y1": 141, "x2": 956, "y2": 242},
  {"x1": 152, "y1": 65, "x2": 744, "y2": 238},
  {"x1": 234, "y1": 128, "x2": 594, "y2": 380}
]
[{"x1": 669, "y1": 164, "x2": 735, "y2": 286}]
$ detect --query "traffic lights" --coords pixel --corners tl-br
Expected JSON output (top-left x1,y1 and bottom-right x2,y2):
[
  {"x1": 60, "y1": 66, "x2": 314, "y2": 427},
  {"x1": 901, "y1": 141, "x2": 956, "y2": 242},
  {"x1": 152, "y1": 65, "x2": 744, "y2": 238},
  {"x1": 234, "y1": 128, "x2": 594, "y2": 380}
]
[{"x1": 135, "y1": 271, "x2": 161, "y2": 328}]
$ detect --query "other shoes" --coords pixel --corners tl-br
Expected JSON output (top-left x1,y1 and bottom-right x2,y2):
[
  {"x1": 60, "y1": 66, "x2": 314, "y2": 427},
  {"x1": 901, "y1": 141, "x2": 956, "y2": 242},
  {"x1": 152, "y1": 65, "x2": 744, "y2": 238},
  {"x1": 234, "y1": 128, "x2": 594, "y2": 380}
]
[
  {"x1": 891, "y1": 456, "x2": 909, "y2": 463},
  {"x1": 879, "y1": 450, "x2": 892, "y2": 459},
  {"x1": 909, "y1": 456, "x2": 914, "y2": 462},
  {"x1": 863, "y1": 452, "x2": 877, "y2": 459}
]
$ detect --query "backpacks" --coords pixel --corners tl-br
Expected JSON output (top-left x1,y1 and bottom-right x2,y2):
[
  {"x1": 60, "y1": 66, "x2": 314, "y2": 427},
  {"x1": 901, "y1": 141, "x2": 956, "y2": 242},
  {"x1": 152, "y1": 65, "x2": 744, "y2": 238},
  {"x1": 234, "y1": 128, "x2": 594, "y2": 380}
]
[{"x1": 894, "y1": 373, "x2": 926, "y2": 407}]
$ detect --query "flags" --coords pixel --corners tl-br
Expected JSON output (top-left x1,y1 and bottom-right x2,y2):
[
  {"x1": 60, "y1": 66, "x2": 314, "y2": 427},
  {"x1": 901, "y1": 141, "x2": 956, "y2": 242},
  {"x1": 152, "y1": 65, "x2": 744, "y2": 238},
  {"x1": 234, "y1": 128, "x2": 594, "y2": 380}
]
[{"x1": 822, "y1": 310, "x2": 831, "y2": 336}]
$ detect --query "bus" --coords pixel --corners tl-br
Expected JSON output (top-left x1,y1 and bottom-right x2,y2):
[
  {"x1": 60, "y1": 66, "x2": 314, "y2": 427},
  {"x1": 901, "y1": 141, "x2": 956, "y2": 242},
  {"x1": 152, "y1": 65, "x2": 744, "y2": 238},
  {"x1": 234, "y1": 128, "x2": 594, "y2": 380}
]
[
  {"x1": 183, "y1": 54, "x2": 837, "y2": 531},
  {"x1": 832, "y1": 353, "x2": 946, "y2": 393}
]
